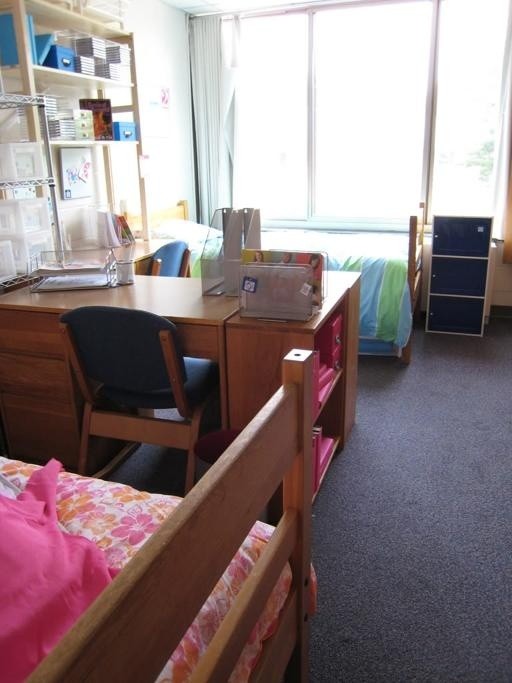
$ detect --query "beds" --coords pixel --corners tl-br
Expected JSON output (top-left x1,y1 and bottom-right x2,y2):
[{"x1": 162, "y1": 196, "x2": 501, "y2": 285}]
[
  {"x1": 118, "y1": 199, "x2": 428, "y2": 365},
  {"x1": 0, "y1": 347, "x2": 318, "y2": 683}
]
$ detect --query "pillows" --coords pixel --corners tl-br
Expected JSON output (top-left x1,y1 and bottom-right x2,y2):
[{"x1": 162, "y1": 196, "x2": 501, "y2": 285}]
[{"x1": 158, "y1": 219, "x2": 222, "y2": 247}]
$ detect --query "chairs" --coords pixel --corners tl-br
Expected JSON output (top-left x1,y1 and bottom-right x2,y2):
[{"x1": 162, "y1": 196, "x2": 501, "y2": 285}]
[
  {"x1": 57, "y1": 304, "x2": 219, "y2": 497},
  {"x1": 148, "y1": 238, "x2": 194, "y2": 277}
]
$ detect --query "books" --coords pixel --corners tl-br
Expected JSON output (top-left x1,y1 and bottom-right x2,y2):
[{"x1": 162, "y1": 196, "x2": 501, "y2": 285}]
[{"x1": 38, "y1": 263, "x2": 107, "y2": 273}]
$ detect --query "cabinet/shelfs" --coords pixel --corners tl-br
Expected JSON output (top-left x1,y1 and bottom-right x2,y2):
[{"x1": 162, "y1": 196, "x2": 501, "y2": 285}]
[
  {"x1": 0, "y1": 0, "x2": 174, "y2": 293},
  {"x1": 422, "y1": 213, "x2": 496, "y2": 339},
  {"x1": 223, "y1": 271, "x2": 361, "y2": 511}
]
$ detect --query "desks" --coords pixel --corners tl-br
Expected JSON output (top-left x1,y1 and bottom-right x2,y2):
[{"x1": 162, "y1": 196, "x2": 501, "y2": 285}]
[
  {"x1": 0, "y1": 266, "x2": 241, "y2": 516},
  {"x1": 73, "y1": 240, "x2": 162, "y2": 275}
]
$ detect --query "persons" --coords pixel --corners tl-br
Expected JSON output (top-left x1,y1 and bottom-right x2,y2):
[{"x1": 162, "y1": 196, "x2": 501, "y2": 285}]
[
  {"x1": 254, "y1": 252, "x2": 265, "y2": 262},
  {"x1": 280, "y1": 253, "x2": 292, "y2": 265},
  {"x1": 308, "y1": 255, "x2": 321, "y2": 269}
]
